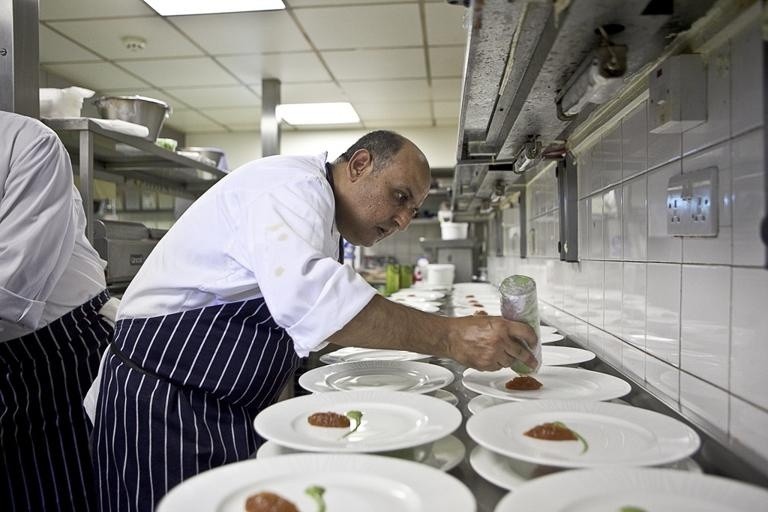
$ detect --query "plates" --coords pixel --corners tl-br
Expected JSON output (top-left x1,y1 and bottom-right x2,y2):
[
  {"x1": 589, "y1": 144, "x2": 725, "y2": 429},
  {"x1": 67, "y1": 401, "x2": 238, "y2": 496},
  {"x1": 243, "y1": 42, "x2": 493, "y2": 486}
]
[
  {"x1": 493, "y1": 464, "x2": 768, "y2": 511},
  {"x1": 154, "y1": 453, "x2": 479, "y2": 511},
  {"x1": 319, "y1": 279, "x2": 598, "y2": 369},
  {"x1": 299, "y1": 359, "x2": 456, "y2": 394},
  {"x1": 459, "y1": 364, "x2": 633, "y2": 402},
  {"x1": 253, "y1": 388, "x2": 466, "y2": 478},
  {"x1": 468, "y1": 401, "x2": 702, "y2": 494}
]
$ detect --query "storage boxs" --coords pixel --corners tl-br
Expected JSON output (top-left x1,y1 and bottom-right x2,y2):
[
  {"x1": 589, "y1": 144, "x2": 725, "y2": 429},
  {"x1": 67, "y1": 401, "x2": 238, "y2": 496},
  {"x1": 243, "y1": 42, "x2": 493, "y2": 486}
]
[{"x1": 38, "y1": 87, "x2": 84, "y2": 120}]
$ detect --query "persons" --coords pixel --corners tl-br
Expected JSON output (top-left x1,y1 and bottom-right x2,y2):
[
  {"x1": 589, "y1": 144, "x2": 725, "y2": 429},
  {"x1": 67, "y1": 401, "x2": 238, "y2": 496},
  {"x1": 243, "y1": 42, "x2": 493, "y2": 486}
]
[
  {"x1": 0, "y1": 109, "x2": 118, "y2": 512},
  {"x1": 81, "y1": 129, "x2": 540, "y2": 512}
]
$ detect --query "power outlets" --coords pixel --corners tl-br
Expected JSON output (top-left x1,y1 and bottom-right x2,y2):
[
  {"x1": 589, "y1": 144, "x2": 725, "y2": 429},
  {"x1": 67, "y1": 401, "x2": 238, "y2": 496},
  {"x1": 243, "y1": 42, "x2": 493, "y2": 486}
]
[{"x1": 667, "y1": 165, "x2": 719, "y2": 235}]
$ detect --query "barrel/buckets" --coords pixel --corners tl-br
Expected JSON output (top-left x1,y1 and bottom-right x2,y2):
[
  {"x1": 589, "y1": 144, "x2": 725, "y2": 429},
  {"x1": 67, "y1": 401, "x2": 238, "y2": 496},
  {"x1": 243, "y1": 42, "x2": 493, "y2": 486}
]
[{"x1": 424, "y1": 263, "x2": 455, "y2": 287}]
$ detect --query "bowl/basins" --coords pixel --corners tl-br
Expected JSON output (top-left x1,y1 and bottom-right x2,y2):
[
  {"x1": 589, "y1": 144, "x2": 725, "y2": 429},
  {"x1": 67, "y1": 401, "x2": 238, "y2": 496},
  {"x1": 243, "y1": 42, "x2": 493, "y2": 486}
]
[
  {"x1": 179, "y1": 145, "x2": 225, "y2": 167},
  {"x1": 92, "y1": 95, "x2": 171, "y2": 142}
]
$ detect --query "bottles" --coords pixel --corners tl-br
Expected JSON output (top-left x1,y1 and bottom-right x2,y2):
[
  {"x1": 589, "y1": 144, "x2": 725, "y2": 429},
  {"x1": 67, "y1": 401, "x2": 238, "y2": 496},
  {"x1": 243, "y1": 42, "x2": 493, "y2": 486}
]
[{"x1": 499, "y1": 274, "x2": 544, "y2": 378}]
